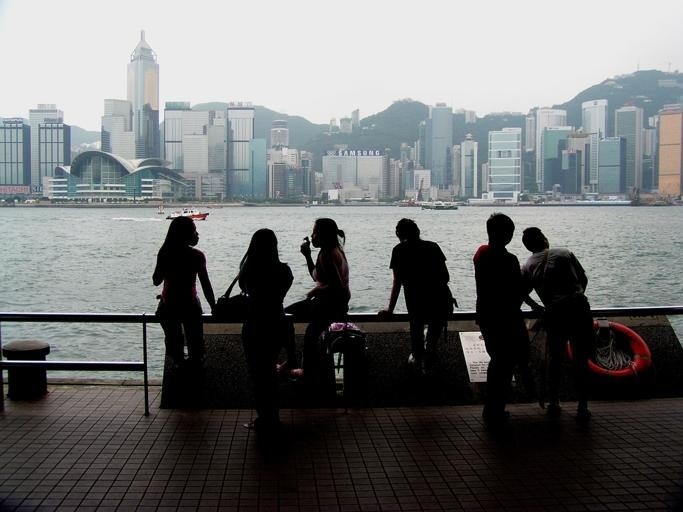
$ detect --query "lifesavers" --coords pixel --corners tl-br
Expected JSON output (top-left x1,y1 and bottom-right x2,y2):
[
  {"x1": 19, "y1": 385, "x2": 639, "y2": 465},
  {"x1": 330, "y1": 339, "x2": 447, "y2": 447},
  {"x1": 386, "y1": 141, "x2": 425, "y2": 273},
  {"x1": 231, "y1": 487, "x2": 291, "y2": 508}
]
[{"x1": 564, "y1": 320, "x2": 651, "y2": 377}]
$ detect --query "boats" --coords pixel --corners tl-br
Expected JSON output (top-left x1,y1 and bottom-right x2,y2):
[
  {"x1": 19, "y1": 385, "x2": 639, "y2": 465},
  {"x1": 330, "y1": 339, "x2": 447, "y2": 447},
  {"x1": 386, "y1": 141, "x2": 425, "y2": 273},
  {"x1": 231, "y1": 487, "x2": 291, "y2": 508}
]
[
  {"x1": 632, "y1": 194, "x2": 672, "y2": 206},
  {"x1": 165, "y1": 208, "x2": 209, "y2": 221},
  {"x1": 395, "y1": 199, "x2": 458, "y2": 210}
]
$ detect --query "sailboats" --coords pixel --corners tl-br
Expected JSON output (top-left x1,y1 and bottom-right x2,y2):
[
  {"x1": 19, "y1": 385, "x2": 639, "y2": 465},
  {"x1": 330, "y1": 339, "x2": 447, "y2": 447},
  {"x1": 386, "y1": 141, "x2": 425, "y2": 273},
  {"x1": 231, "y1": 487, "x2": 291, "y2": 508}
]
[{"x1": 157, "y1": 205, "x2": 164, "y2": 214}]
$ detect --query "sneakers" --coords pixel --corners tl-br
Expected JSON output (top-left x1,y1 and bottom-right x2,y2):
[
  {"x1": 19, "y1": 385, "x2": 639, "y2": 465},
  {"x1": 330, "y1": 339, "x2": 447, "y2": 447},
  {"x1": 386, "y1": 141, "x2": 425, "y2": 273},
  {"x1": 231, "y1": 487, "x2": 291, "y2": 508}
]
[
  {"x1": 546, "y1": 405, "x2": 560, "y2": 417},
  {"x1": 576, "y1": 408, "x2": 590, "y2": 421},
  {"x1": 481, "y1": 405, "x2": 509, "y2": 420}
]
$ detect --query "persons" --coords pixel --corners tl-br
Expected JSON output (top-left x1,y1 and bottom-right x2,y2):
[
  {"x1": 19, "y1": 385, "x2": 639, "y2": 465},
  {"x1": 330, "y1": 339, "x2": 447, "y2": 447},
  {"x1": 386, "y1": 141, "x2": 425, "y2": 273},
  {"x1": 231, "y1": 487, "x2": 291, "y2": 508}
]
[
  {"x1": 284, "y1": 219, "x2": 352, "y2": 385},
  {"x1": 378, "y1": 218, "x2": 454, "y2": 367},
  {"x1": 153, "y1": 217, "x2": 217, "y2": 410},
  {"x1": 237, "y1": 229, "x2": 294, "y2": 430},
  {"x1": 520, "y1": 227, "x2": 593, "y2": 423},
  {"x1": 473, "y1": 213, "x2": 547, "y2": 427}
]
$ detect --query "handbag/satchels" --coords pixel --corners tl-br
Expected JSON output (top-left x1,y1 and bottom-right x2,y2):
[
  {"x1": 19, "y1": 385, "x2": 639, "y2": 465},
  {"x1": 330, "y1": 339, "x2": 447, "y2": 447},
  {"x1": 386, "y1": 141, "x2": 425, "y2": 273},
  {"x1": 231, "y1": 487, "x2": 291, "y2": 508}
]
[{"x1": 213, "y1": 275, "x2": 248, "y2": 322}]
